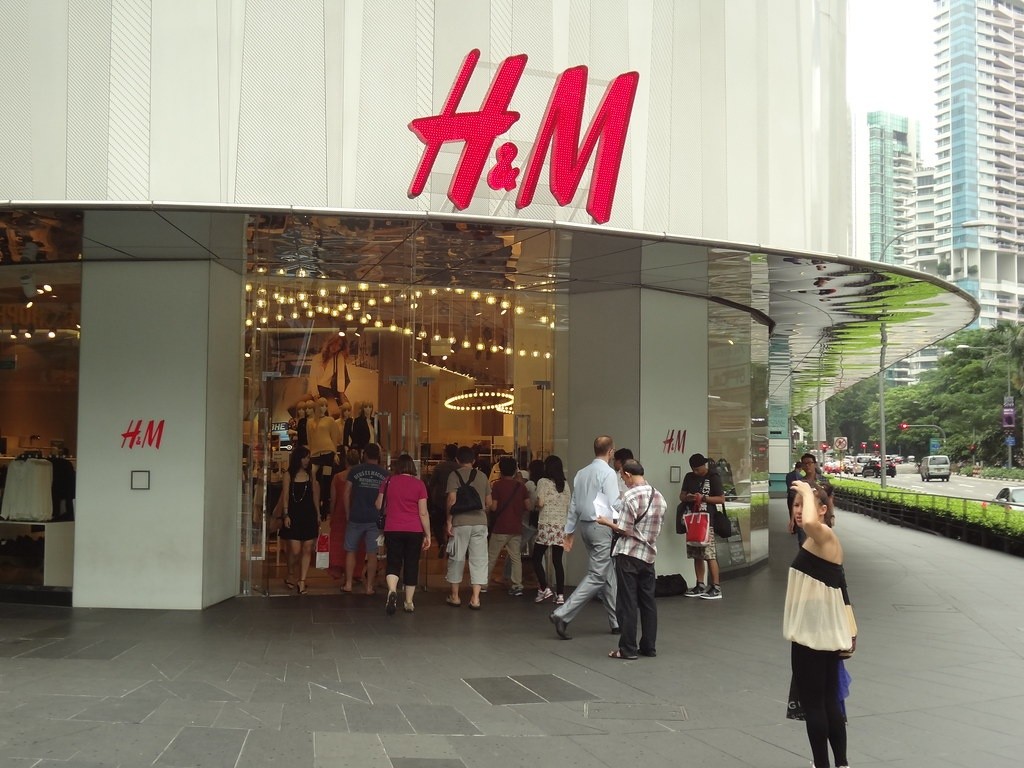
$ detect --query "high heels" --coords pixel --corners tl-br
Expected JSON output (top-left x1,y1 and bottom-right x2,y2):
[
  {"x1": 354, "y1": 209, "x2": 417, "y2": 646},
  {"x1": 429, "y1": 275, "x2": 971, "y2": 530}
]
[
  {"x1": 285, "y1": 573, "x2": 295, "y2": 589},
  {"x1": 297, "y1": 579, "x2": 308, "y2": 596}
]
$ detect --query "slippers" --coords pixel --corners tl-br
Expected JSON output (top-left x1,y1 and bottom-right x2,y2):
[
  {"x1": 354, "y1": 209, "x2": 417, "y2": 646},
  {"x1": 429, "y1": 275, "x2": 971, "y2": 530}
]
[
  {"x1": 366, "y1": 588, "x2": 377, "y2": 597},
  {"x1": 340, "y1": 585, "x2": 352, "y2": 594}
]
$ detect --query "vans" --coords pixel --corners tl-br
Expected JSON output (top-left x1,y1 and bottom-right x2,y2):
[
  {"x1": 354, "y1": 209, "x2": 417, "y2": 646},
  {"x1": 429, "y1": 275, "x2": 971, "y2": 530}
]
[{"x1": 918, "y1": 454, "x2": 951, "y2": 482}]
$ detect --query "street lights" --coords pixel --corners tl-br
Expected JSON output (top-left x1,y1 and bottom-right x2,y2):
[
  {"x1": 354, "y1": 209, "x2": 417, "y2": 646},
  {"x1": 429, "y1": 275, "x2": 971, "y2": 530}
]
[
  {"x1": 878, "y1": 219, "x2": 996, "y2": 490},
  {"x1": 913, "y1": 400, "x2": 936, "y2": 438},
  {"x1": 956, "y1": 344, "x2": 1012, "y2": 472}
]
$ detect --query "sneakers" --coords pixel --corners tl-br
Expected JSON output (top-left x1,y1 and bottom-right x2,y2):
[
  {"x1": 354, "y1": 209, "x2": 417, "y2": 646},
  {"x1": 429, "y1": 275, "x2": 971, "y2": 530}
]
[
  {"x1": 385, "y1": 590, "x2": 398, "y2": 615},
  {"x1": 700, "y1": 584, "x2": 722, "y2": 600},
  {"x1": 447, "y1": 580, "x2": 565, "y2": 607},
  {"x1": 403, "y1": 600, "x2": 414, "y2": 612},
  {"x1": 685, "y1": 583, "x2": 706, "y2": 597}
]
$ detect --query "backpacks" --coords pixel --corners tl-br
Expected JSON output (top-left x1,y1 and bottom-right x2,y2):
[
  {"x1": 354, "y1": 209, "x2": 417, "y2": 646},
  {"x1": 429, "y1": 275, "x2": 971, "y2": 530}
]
[{"x1": 450, "y1": 468, "x2": 483, "y2": 514}]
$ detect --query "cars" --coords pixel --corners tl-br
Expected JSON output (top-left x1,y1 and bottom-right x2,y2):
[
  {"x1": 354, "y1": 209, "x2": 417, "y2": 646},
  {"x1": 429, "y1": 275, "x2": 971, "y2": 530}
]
[
  {"x1": 810, "y1": 449, "x2": 915, "y2": 478},
  {"x1": 990, "y1": 486, "x2": 1024, "y2": 512}
]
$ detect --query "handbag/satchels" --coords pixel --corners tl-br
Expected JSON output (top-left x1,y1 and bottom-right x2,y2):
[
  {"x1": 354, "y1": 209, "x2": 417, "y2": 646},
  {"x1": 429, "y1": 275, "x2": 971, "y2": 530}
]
[
  {"x1": 838, "y1": 637, "x2": 856, "y2": 659},
  {"x1": 376, "y1": 515, "x2": 386, "y2": 530},
  {"x1": 675, "y1": 503, "x2": 733, "y2": 548},
  {"x1": 330, "y1": 354, "x2": 350, "y2": 396},
  {"x1": 313, "y1": 526, "x2": 330, "y2": 570},
  {"x1": 610, "y1": 532, "x2": 621, "y2": 557},
  {"x1": 268, "y1": 492, "x2": 284, "y2": 533},
  {"x1": 653, "y1": 574, "x2": 688, "y2": 597}
]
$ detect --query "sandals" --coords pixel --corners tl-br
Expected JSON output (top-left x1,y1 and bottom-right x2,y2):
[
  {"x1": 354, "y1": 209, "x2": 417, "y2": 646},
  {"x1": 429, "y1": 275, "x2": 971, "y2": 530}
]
[{"x1": 609, "y1": 650, "x2": 638, "y2": 659}]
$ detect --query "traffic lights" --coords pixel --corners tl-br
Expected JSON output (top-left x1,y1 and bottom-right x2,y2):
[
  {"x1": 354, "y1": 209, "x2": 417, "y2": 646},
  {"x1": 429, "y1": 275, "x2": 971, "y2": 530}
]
[
  {"x1": 898, "y1": 423, "x2": 907, "y2": 429},
  {"x1": 970, "y1": 445, "x2": 976, "y2": 455}
]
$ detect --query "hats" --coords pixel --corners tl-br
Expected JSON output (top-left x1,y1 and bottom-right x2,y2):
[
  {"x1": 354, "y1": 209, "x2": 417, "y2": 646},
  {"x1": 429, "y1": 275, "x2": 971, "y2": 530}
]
[{"x1": 689, "y1": 454, "x2": 709, "y2": 468}]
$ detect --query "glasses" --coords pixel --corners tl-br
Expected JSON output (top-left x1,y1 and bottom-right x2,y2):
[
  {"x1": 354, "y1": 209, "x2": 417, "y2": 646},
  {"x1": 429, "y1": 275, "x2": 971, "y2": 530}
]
[
  {"x1": 801, "y1": 461, "x2": 815, "y2": 468},
  {"x1": 620, "y1": 471, "x2": 628, "y2": 481}
]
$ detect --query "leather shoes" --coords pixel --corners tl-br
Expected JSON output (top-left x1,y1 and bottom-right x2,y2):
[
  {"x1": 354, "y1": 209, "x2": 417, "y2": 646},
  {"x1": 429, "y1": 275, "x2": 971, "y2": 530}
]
[
  {"x1": 612, "y1": 627, "x2": 621, "y2": 634},
  {"x1": 549, "y1": 612, "x2": 573, "y2": 639}
]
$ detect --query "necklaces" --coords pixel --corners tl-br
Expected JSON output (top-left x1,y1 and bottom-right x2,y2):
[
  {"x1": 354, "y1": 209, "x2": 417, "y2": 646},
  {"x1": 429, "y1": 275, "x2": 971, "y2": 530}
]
[{"x1": 292, "y1": 473, "x2": 308, "y2": 502}]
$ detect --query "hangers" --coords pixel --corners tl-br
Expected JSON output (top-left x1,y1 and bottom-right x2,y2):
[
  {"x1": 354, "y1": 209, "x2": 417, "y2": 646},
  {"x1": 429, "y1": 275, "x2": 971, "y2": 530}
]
[{"x1": 9, "y1": 449, "x2": 74, "y2": 467}]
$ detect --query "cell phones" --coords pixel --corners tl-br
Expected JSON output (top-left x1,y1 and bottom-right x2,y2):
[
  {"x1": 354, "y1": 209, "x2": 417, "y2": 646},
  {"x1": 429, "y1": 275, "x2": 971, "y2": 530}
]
[{"x1": 690, "y1": 495, "x2": 696, "y2": 497}]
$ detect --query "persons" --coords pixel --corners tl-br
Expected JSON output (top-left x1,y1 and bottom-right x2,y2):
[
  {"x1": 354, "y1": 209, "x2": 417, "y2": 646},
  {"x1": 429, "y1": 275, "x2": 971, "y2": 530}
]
[
  {"x1": 782, "y1": 480, "x2": 858, "y2": 768},
  {"x1": 429, "y1": 435, "x2": 668, "y2": 661},
  {"x1": 306, "y1": 331, "x2": 352, "y2": 419},
  {"x1": 789, "y1": 453, "x2": 834, "y2": 555},
  {"x1": 288, "y1": 397, "x2": 381, "y2": 519},
  {"x1": 786, "y1": 462, "x2": 828, "y2": 534},
  {"x1": 279, "y1": 447, "x2": 322, "y2": 595},
  {"x1": 329, "y1": 443, "x2": 431, "y2": 615},
  {"x1": 679, "y1": 454, "x2": 724, "y2": 600}
]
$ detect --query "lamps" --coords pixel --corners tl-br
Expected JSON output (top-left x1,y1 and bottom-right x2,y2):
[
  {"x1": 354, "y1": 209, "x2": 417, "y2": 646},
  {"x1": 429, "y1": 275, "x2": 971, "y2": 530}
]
[
  {"x1": 49, "y1": 328, "x2": 57, "y2": 338},
  {"x1": 415, "y1": 323, "x2": 423, "y2": 341},
  {"x1": 422, "y1": 344, "x2": 430, "y2": 356},
  {"x1": 483, "y1": 327, "x2": 493, "y2": 343},
  {"x1": 25, "y1": 328, "x2": 35, "y2": 339},
  {"x1": 450, "y1": 341, "x2": 459, "y2": 354},
  {"x1": 499, "y1": 336, "x2": 506, "y2": 350},
  {"x1": 355, "y1": 325, "x2": 364, "y2": 337},
  {"x1": 339, "y1": 325, "x2": 347, "y2": 336},
  {"x1": 11, "y1": 327, "x2": 19, "y2": 339},
  {"x1": 19, "y1": 294, "x2": 34, "y2": 308}
]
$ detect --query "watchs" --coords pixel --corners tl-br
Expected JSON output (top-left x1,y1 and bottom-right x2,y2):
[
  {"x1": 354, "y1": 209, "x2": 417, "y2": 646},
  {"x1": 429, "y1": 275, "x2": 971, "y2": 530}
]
[{"x1": 702, "y1": 495, "x2": 706, "y2": 502}]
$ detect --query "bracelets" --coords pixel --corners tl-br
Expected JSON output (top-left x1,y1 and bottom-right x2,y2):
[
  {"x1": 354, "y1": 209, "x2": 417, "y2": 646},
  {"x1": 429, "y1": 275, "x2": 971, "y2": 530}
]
[
  {"x1": 830, "y1": 515, "x2": 835, "y2": 518},
  {"x1": 283, "y1": 513, "x2": 289, "y2": 518}
]
